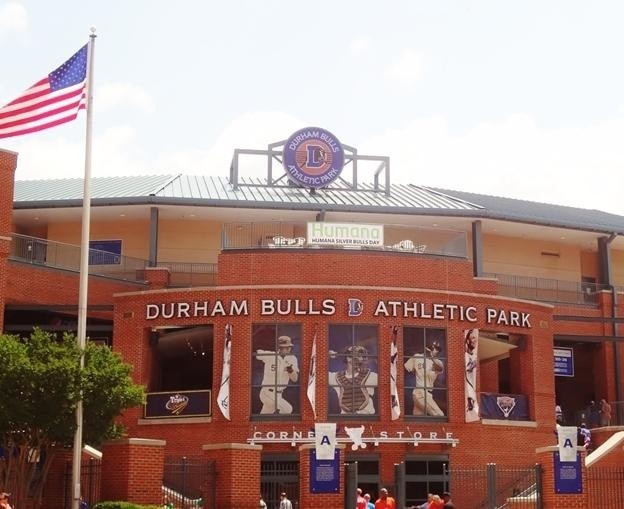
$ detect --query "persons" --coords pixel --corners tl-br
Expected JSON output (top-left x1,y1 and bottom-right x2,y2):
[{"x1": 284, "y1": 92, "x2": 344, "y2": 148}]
[
  {"x1": 329, "y1": 345, "x2": 378, "y2": 414},
  {"x1": 259, "y1": 495, "x2": 267, "y2": 509},
  {"x1": 555, "y1": 402, "x2": 565, "y2": 425},
  {"x1": 418, "y1": 493, "x2": 433, "y2": 509},
  {"x1": 162, "y1": 495, "x2": 176, "y2": 509},
  {"x1": 364, "y1": 493, "x2": 374, "y2": 509},
  {"x1": 577, "y1": 398, "x2": 611, "y2": 448},
  {"x1": 252, "y1": 335, "x2": 300, "y2": 414},
  {"x1": 197, "y1": 498, "x2": 206, "y2": 509},
  {"x1": 0, "y1": 492, "x2": 12, "y2": 509},
  {"x1": 404, "y1": 339, "x2": 446, "y2": 415},
  {"x1": 356, "y1": 488, "x2": 367, "y2": 509},
  {"x1": 80, "y1": 496, "x2": 88, "y2": 508},
  {"x1": 441, "y1": 491, "x2": 456, "y2": 509},
  {"x1": 279, "y1": 492, "x2": 293, "y2": 509},
  {"x1": 513, "y1": 485, "x2": 520, "y2": 496},
  {"x1": 465, "y1": 329, "x2": 477, "y2": 420},
  {"x1": 375, "y1": 488, "x2": 397, "y2": 509},
  {"x1": 390, "y1": 326, "x2": 398, "y2": 412}
]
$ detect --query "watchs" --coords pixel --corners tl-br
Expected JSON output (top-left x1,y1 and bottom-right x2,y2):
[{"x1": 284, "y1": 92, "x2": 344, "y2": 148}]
[{"x1": 428, "y1": 494, "x2": 452, "y2": 509}]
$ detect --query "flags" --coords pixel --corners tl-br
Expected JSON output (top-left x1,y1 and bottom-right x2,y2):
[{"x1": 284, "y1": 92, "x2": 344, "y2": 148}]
[
  {"x1": 216, "y1": 323, "x2": 235, "y2": 421},
  {"x1": 306, "y1": 331, "x2": 318, "y2": 421},
  {"x1": 0, "y1": 41, "x2": 88, "y2": 138},
  {"x1": 387, "y1": 324, "x2": 402, "y2": 421},
  {"x1": 463, "y1": 327, "x2": 481, "y2": 422}
]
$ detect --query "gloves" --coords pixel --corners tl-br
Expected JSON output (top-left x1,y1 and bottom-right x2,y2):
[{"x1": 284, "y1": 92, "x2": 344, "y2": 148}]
[{"x1": 287, "y1": 365, "x2": 293, "y2": 373}]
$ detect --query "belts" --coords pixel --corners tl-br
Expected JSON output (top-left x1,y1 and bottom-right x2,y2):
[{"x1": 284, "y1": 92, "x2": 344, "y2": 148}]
[{"x1": 269, "y1": 389, "x2": 281, "y2": 393}]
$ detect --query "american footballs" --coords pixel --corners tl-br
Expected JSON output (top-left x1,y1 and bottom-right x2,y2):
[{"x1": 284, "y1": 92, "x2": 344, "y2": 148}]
[{"x1": 168, "y1": 396, "x2": 186, "y2": 410}]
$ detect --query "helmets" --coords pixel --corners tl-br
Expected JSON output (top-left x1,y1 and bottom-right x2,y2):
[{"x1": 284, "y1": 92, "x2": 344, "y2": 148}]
[
  {"x1": 344, "y1": 346, "x2": 368, "y2": 374},
  {"x1": 278, "y1": 336, "x2": 295, "y2": 348}
]
